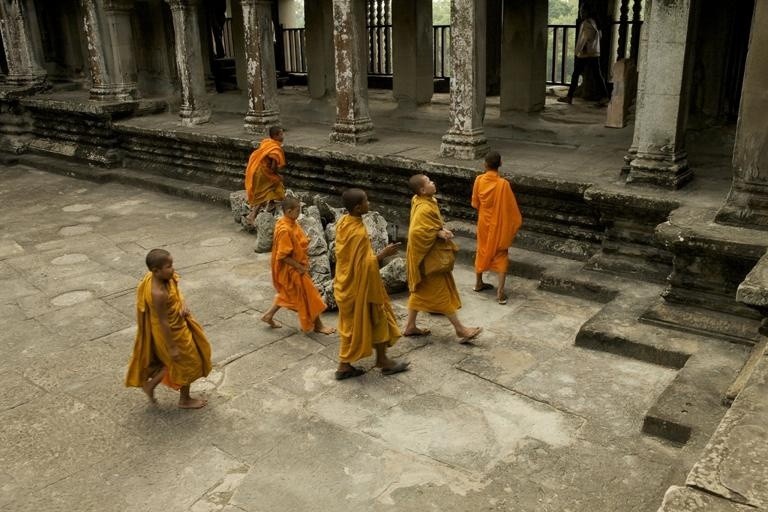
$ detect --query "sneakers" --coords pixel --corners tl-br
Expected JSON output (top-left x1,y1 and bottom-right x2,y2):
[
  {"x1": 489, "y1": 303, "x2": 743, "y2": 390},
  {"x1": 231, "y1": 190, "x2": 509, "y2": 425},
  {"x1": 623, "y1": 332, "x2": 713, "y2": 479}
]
[{"x1": 558, "y1": 97, "x2": 609, "y2": 107}]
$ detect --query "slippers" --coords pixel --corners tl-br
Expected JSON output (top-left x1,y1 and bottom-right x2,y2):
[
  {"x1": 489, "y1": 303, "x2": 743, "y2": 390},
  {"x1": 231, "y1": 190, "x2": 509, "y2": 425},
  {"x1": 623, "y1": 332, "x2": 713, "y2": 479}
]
[
  {"x1": 383, "y1": 360, "x2": 410, "y2": 375},
  {"x1": 335, "y1": 367, "x2": 363, "y2": 379},
  {"x1": 460, "y1": 328, "x2": 480, "y2": 343},
  {"x1": 405, "y1": 328, "x2": 431, "y2": 336},
  {"x1": 498, "y1": 299, "x2": 507, "y2": 304},
  {"x1": 474, "y1": 284, "x2": 493, "y2": 291}
]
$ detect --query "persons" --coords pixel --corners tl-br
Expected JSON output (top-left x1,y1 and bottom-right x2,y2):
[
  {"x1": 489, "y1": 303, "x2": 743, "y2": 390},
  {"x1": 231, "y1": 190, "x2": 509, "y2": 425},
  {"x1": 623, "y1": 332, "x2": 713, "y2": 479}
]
[
  {"x1": 469, "y1": 149, "x2": 523, "y2": 310},
  {"x1": 240, "y1": 124, "x2": 290, "y2": 235},
  {"x1": 125, "y1": 251, "x2": 213, "y2": 410},
  {"x1": 402, "y1": 173, "x2": 479, "y2": 343},
  {"x1": 330, "y1": 188, "x2": 404, "y2": 378},
  {"x1": 559, "y1": 9, "x2": 610, "y2": 109},
  {"x1": 260, "y1": 193, "x2": 337, "y2": 336}
]
